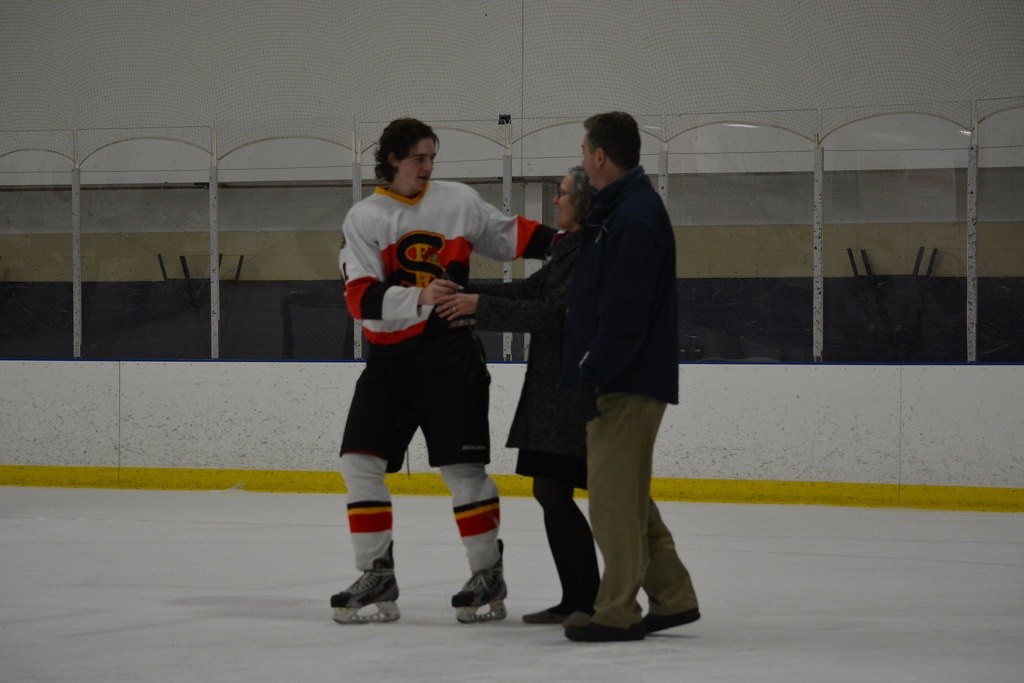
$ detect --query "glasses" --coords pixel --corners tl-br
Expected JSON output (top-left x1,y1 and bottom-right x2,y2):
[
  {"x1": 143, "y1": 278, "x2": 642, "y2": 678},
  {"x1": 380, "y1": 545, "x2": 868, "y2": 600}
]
[{"x1": 557, "y1": 189, "x2": 570, "y2": 197}]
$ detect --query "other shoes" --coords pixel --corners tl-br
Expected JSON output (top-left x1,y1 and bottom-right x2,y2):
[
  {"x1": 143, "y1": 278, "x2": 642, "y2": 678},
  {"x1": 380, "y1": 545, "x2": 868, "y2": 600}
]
[
  {"x1": 641, "y1": 608, "x2": 699, "y2": 634},
  {"x1": 522, "y1": 607, "x2": 569, "y2": 625},
  {"x1": 566, "y1": 621, "x2": 642, "y2": 643}
]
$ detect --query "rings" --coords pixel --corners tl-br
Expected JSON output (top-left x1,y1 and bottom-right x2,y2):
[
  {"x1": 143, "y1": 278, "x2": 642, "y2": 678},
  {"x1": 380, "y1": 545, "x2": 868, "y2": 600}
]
[{"x1": 453, "y1": 306, "x2": 456, "y2": 313}]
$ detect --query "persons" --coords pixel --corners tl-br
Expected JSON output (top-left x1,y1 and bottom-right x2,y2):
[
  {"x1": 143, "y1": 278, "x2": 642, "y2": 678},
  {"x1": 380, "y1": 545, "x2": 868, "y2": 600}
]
[
  {"x1": 430, "y1": 167, "x2": 601, "y2": 625},
  {"x1": 327, "y1": 118, "x2": 561, "y2": 624},
  {"x1": 563, "y1": 111, "x2": 703, "y2": 644}
]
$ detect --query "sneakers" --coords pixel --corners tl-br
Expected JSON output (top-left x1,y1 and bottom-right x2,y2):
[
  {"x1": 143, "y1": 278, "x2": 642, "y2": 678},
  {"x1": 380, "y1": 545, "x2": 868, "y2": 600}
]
[
  {"x1": 331, "y1": 540, "x2": 401, "y2": 624},
  {"x1": 452, "y1": 538, "x2": 507, "y2": 623}
]
